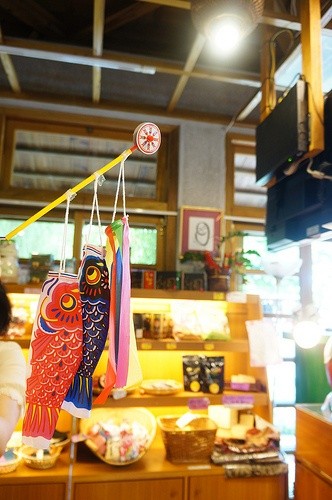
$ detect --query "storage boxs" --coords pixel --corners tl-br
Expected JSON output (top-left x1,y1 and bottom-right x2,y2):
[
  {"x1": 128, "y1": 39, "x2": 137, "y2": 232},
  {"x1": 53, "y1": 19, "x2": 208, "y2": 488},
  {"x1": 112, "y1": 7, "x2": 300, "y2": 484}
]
[
  {"x1": 183, "y1": 271, "x2": 208, "y2": 290},
  {"x1": 207, "y1": 404, "x2": 253, "y2": 430},
  {"x1": 133, "y1": 312, "x2": 154, "y2": 339},
  {"x1": 209, "y1": 275, "x2": 231, "y2": 291},
  {"x1": 130, "y1": 269, "x2": 157, "y2": 289},
  {"x1": 154, "y1": 314, "x2": 174, "y2": 339},
  {"x1": 230, "y1": 383, "x2": 263, "y2": 392},
  {"x1": 156, "y1": 271, "x2": 181, "y2": 290},
  {"x1": 28, "y1": 254, "x2": 55, "y2": 285}
]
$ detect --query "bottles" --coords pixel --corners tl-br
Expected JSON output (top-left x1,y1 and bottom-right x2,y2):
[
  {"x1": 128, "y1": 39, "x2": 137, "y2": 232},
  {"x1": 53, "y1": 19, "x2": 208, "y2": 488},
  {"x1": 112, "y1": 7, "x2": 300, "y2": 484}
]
[
  {"x1": 175, "y1": 397, "x2": 210, "y2": 430},
  {"x1": 133, "y1": 313, "x2": 168, "y2": 339},
  {"x1": 0, "y1": 239, "x2": 20, "y2": 283}
]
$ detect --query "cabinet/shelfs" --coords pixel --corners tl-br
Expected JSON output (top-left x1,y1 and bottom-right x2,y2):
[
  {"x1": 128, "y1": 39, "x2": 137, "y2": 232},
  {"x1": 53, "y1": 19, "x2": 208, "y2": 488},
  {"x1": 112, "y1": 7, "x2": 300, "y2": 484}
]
[
  {"x1": 294, "y1": 403, "x2": 332, "y2": 500},
  {"x1": 0, "y1": 283, "x2": 289, "y2": 500}
]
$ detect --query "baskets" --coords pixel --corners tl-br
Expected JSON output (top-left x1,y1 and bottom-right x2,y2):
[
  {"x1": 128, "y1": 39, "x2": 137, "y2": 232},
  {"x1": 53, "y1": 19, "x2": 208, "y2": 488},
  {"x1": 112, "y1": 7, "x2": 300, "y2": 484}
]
[
  {"x1": 157, "y1": 414, "x2": 219, "y2": 464},
  {"x1": 79, "y1": 406, "x2": 158, "y2": 467},
  {"x1": 0, "y1": 456, "x2": 23, "y2": 474},
  {"x1": 16, "y1": 443, "x2": 63, "y2": 470}
]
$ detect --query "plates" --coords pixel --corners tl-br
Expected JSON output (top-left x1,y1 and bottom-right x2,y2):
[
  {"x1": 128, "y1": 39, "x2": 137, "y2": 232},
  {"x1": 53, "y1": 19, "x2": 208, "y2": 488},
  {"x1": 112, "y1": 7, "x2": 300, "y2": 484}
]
[{"x1": 140, "y1": 380, "x2": 181, "y2": 395}]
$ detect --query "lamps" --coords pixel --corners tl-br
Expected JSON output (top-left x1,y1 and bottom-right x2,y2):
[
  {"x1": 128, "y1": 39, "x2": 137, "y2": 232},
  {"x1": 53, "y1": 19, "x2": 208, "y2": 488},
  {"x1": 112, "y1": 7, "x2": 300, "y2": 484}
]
[{"x1": 190, "y1": 0, "x2": 265, "y2": 68}]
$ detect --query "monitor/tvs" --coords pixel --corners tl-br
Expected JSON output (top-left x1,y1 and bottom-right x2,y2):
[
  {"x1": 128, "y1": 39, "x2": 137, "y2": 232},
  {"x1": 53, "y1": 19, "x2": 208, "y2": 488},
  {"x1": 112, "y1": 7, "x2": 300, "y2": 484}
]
[{"x1": 255, "y1": 80, "x2": 332, "y2": 252}]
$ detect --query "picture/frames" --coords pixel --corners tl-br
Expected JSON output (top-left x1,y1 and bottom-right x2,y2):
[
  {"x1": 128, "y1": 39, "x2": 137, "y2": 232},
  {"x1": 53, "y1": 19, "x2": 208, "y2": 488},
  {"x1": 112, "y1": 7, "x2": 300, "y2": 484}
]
[{"x1": 177, "y1": 205, "x2": 225, "y2": 261}]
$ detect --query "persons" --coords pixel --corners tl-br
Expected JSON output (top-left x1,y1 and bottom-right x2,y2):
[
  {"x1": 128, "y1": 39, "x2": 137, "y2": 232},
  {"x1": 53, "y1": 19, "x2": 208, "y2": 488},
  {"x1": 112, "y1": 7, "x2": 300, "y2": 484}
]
[{"x1": 0, "y1": 279, "x2": 26, "y2": 458}]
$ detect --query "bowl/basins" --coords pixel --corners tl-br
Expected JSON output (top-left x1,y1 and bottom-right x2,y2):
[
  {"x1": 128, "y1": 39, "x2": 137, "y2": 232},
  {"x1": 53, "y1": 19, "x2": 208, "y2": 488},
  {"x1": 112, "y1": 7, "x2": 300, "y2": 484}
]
[{"x1": 19, "y1": 446, "x2": 61, "y2": 469}]
